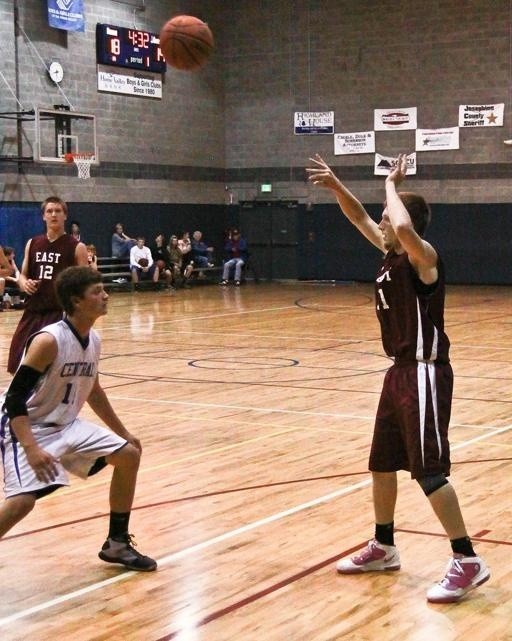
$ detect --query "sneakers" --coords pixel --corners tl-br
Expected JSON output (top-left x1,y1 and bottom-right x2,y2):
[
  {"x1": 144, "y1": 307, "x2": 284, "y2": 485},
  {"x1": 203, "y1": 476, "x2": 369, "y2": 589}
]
[
  {"x1": 427, "y1": 557, "x2": 490, "y2": 603},
  {"x1": 219, "y1": 280, "x2": 228, "y2": 285},
  {"x1": 99, "y1": 534, "x2": 157, "y2": 572},
  {"x1": 336, "y1": 540, "x2": 400, "y2": 574}
]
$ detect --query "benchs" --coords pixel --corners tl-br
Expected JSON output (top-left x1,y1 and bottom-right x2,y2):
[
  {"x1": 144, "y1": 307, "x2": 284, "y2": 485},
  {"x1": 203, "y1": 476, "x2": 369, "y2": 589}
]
[{"x1": 97, "y1": 251, "x2": 225, "y2": 289}]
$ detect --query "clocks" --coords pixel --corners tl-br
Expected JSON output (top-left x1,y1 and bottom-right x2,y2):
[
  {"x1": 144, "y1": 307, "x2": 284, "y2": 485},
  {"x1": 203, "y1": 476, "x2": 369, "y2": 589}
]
[{"x1": 47, "y1": 57, "x2": 65, "y2": 84}]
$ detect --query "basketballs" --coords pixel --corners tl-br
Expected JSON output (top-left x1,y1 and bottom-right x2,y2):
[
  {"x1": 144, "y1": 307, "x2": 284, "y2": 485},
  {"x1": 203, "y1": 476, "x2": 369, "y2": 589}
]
[
  {"x1": 156, "y1": 260, "x2": 165, "y2": 270},
  {"x1": 159, "y1": 15, "x2": 212, "y2": 70},
  {"x1": 138, "y1": 258, "x2": 148, "y2": 267}
]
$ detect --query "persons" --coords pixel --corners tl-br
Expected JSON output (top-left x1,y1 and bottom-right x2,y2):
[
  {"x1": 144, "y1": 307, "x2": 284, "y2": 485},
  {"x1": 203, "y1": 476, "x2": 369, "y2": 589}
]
[
  {"x1": 1, "y1": 264, "x2": 159, "y2": 574},
  {"x1": 1, "y1": 220, "x2": 251, "y2": 312},
  {"x1": 304, "y1": 152, "x2": 494, "y2": 606},
  {"x1": 7, "y1": 196, "x2": 91, "y2": 380}
]
